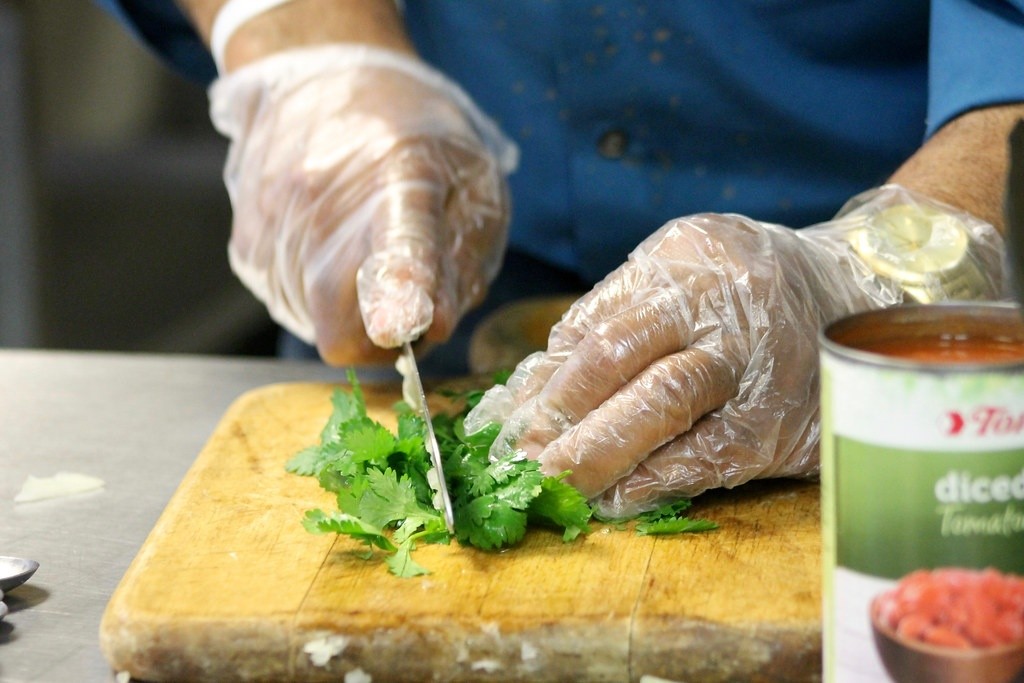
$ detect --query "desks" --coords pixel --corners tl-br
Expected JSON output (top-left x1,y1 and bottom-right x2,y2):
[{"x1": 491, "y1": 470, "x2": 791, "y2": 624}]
[{"x1": 0, "y1": 347, "x2": 399, "y2": 683}]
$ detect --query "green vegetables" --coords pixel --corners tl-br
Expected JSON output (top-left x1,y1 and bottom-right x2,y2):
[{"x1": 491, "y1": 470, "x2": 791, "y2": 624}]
[{"x1": 281, "y1": 363, "x2": 725, "y2": 580}]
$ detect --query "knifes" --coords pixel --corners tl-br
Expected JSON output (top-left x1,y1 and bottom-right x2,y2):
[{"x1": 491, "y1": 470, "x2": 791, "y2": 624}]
[{"x1": 395, "y1": 335, "x2": 453, "y2": 525}]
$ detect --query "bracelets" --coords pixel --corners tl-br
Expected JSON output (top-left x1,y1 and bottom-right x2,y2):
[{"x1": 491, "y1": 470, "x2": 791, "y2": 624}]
[{"x1": 209, "y1": 0, "x2": 289, "y2": 77}]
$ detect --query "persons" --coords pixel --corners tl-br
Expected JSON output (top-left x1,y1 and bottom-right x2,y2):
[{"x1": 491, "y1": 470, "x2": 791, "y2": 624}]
[{"x1": 93, "y1": 0, "x2": 1024, "y2": 521}]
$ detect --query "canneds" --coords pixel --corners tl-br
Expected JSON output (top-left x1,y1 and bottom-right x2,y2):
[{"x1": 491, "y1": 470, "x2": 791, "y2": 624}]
[{"x1": 816, "y1": 298, "x2": 1024, "y2": 683}]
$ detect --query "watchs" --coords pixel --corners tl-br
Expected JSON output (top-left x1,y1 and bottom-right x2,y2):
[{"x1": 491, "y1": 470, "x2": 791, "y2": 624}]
[{"x1": 840, "y1": 204, "x2": 989, "y2": 304}]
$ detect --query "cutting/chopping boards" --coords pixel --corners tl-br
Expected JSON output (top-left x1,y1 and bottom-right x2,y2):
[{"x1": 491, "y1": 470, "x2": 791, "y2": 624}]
[{"x1": 100, "y1": 382, "x2": 821, "y2": 683}]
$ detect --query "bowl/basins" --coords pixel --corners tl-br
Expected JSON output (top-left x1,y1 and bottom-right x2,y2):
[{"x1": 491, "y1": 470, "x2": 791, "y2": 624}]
[{"x1": 870, "y1": 592, "x2": 1024, "y2": 683}]
